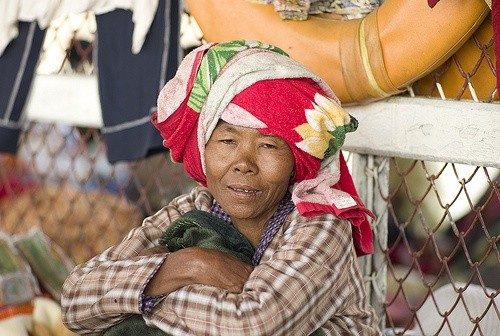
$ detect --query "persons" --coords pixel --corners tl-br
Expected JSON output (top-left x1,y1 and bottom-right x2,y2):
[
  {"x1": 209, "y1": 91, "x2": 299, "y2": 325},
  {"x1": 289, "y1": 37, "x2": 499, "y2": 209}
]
[{"x1": 60, "y1": 40, "x2": 382, "y2": 336}]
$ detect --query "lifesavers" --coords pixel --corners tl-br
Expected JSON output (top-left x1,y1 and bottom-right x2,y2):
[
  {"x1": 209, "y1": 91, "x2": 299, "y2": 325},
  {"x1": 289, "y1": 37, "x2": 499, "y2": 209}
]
[{"x1": 184, "y1": 0, "x2": 492, "y2": 107}]
